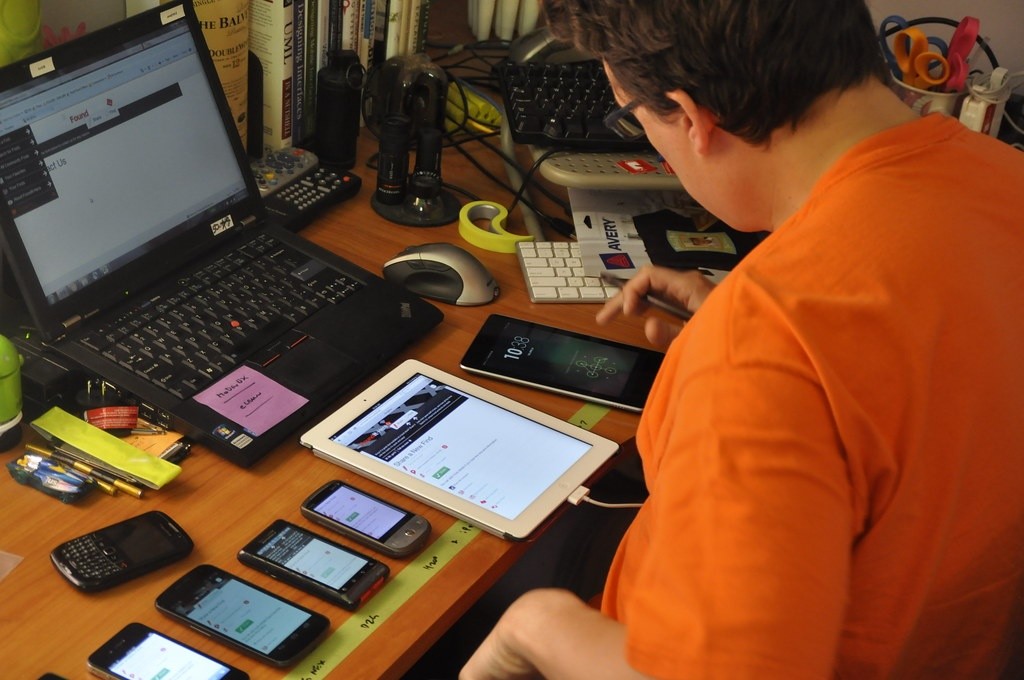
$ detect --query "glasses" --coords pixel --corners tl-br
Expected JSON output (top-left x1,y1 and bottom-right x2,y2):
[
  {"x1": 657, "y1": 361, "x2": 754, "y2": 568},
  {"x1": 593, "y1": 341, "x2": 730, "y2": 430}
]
[{"x1": 604, "y1": 89, "x2": 722, "y2": 141}]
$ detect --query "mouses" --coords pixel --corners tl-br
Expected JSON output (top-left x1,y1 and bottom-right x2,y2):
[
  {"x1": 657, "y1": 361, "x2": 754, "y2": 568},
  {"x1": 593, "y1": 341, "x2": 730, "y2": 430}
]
[{"x1": 383, "y1": 241, "x2": 500, "y2": 305}]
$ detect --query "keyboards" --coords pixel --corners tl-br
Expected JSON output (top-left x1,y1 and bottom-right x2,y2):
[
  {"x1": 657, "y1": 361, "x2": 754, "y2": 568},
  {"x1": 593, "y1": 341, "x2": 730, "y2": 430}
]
[
  {"x1": 514, "y1": 239, "x2": 626, "y2": 304},
  {"x1": 498, "y1": 62, "x2": 656, "y2": 154}
]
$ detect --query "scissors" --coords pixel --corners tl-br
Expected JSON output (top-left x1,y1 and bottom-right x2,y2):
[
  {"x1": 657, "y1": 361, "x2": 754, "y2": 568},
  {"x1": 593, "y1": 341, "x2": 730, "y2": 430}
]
[
  {"x1": 944, "y1": 16, "x2": 980, "y2": 92},
  {"x1": 879, "y1": 15, "x2": 948, "y2": 78},
  {"x1": 893, "y1": 28, "x2": 950, "y2": 89}
]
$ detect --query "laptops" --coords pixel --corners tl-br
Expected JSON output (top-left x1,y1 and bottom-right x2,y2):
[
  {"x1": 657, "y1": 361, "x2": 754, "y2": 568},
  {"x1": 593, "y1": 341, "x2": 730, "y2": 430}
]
[{"x1": 0, "y1": 0, "x2": 443, "y2": 470}]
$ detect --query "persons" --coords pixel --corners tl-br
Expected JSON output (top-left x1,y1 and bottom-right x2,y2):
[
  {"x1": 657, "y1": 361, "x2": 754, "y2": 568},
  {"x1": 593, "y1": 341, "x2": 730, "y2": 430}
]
[{"x1": 456, "y1": 2, "x2": 1023, "y2": 680}]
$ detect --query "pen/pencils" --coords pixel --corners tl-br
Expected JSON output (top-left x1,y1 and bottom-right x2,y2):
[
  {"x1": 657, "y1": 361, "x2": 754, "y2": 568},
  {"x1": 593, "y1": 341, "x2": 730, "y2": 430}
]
[
  {"x1": 600, "y1": 269, "x2": 691, "y2": 318},
  {"x1": 16, "y1": 438, "x2": 144, "y2": 496}
]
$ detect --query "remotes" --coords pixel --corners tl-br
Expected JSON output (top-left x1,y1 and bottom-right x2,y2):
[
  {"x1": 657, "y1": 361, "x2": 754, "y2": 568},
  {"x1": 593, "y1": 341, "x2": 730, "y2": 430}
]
[{"x1": 242, "y1": 145, "x2": 364, "y2": 232}]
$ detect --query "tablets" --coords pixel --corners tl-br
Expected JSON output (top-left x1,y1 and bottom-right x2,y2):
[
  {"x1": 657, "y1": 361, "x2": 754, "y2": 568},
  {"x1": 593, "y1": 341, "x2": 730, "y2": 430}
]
[
  {"x1": 300, "y1": 358, "x2": 620, "y2": 543},
  {"x1": 460, "y1": 314, "x2": 666, "y2": 413}
]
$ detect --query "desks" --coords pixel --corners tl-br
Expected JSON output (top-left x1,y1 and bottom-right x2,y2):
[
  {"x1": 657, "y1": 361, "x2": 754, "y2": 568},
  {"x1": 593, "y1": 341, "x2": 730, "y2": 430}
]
[{"x1": 0, "y1": 127, "x2": 686, "y2": 680}]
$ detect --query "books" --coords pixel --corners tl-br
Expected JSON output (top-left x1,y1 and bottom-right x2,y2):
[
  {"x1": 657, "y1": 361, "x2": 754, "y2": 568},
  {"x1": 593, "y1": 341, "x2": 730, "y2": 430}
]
[{"x1": 126, "y1": 0, "x2": 430, "y2": 163}]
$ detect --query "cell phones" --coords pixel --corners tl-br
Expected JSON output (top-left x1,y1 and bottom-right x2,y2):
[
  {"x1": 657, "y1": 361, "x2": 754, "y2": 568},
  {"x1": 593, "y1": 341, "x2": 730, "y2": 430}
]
[
  {"x1": 299, "y1": 479, "x2": 431, "y2": 560},
  {"x1": 50, "y1": 510, "x2": 193, "y2": 593},
  {"x1": 87, "y1": 622, "x2": 252, "y2": 680},
  {"x1": 155, "y1": 564, "x2": 330, "y2": 668},
  {"x1": 237, "y1": 518, "x2": 390, "y2": 614}
]
